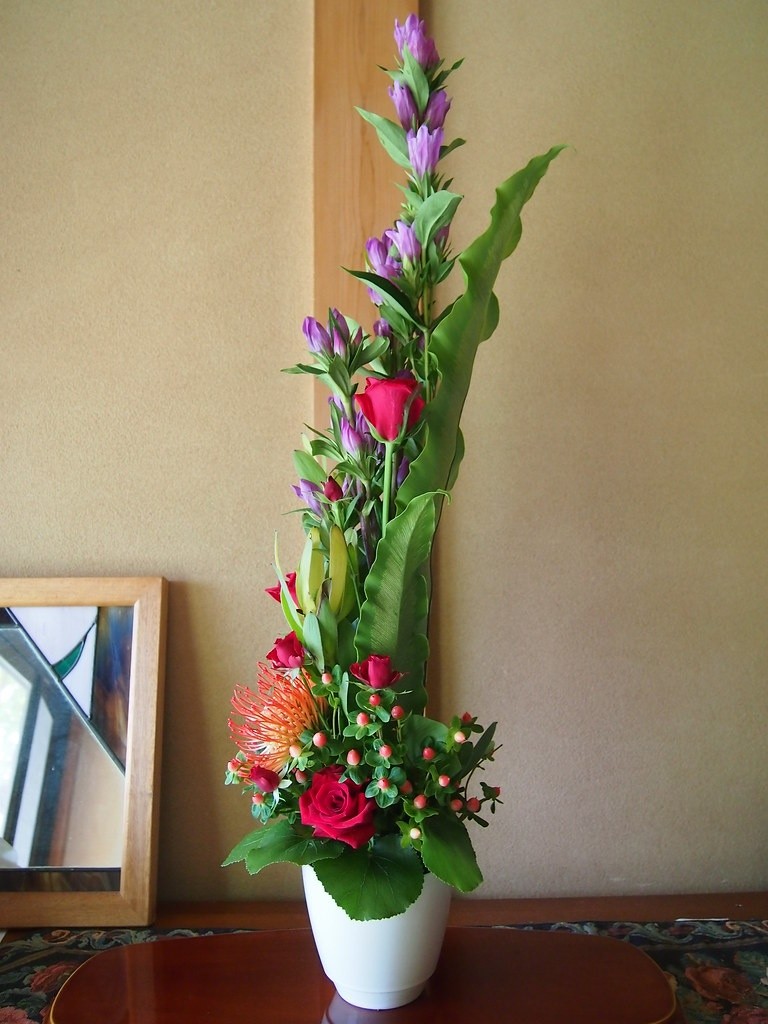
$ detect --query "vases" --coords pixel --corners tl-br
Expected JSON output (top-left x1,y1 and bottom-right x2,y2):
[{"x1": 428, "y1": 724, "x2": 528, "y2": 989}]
[{"x1": 298, "y1": 865, "x2": 451, "y2": 1011}]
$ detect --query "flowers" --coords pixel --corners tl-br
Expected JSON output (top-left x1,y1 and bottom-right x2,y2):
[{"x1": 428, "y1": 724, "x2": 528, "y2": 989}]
[{"x1": 216, "y1": 16, "x2": 576, "y2": 923}]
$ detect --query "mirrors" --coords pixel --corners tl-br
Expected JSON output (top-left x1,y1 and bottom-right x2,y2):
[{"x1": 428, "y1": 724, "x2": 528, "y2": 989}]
[{"x1": 0, "y1": 575, "x2": 170, "y2": 928}]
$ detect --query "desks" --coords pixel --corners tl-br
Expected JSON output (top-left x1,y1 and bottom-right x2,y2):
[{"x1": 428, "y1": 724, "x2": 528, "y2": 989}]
[{"x1": 51, "y1": 931, "x2": 681, "y2": 1024}]
